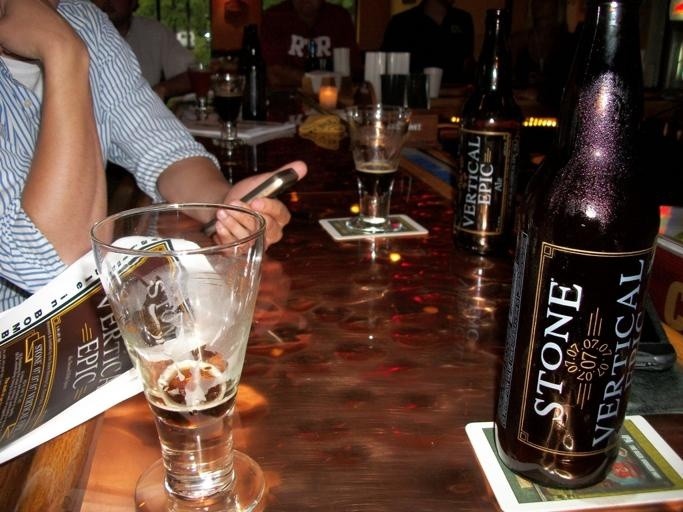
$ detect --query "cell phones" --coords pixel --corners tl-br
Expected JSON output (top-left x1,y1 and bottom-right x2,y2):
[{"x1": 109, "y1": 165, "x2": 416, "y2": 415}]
[{"x1": 203, "y1": 167, "x2": 300, "y2": 239}]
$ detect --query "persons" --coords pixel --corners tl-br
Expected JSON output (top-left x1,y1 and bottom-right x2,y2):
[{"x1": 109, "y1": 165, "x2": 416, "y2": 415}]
[
  {"x1": 379, "y1": 0, "x2": 475, "y2": 88},
  {"x1": 260, "y1": 0, "x2": 352, "y2": 79},
  {"x1": 0, "y1": 1, "x2": 307, "y2": 317},
  {"x1": 516, "y1": 1, "x2": 592, "y2": 113},
  {"x1": 91, "y1": 0, "x2": 201, "y2": 106}
]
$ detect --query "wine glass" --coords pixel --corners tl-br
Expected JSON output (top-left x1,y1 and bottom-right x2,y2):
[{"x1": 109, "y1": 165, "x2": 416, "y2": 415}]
[
  {"x1": 89, "y1": 202, "x2": 266, "y2": 511},
  {"x1": 345, "y1": 104, "x2": 412, "y2": 233},
  {"x1": 210, "y1": 74, "x2": 245, "y2": 150},
  {"x1": 188, "y1": 63, "x2": 213, "y2": 114}
]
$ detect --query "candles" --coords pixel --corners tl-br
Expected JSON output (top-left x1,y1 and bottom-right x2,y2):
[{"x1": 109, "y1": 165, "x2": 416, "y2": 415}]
[{"x1": 319, "y1": 85, "x2": 337, "y2": 110}]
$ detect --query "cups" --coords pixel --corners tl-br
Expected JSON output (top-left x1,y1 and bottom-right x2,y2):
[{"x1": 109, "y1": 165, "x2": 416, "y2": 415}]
[
  {"x1": 380, "y1": 74, "x2": 408, "y2": 110},
  {"x1": 423, "y1": 68, "x2": 443, "y2": 99}
]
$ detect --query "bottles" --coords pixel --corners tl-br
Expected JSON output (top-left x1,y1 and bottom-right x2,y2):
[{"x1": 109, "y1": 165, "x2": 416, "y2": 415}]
[
  {"x1": 242, "y1": 24, "x2": 266, "y2": 122},
  {"x1": 493, "y1": 1, "x2": 660, "y2": 489},
  {"x1": 451, "y1": 8, "x2": 526, "y2": 257}
]
